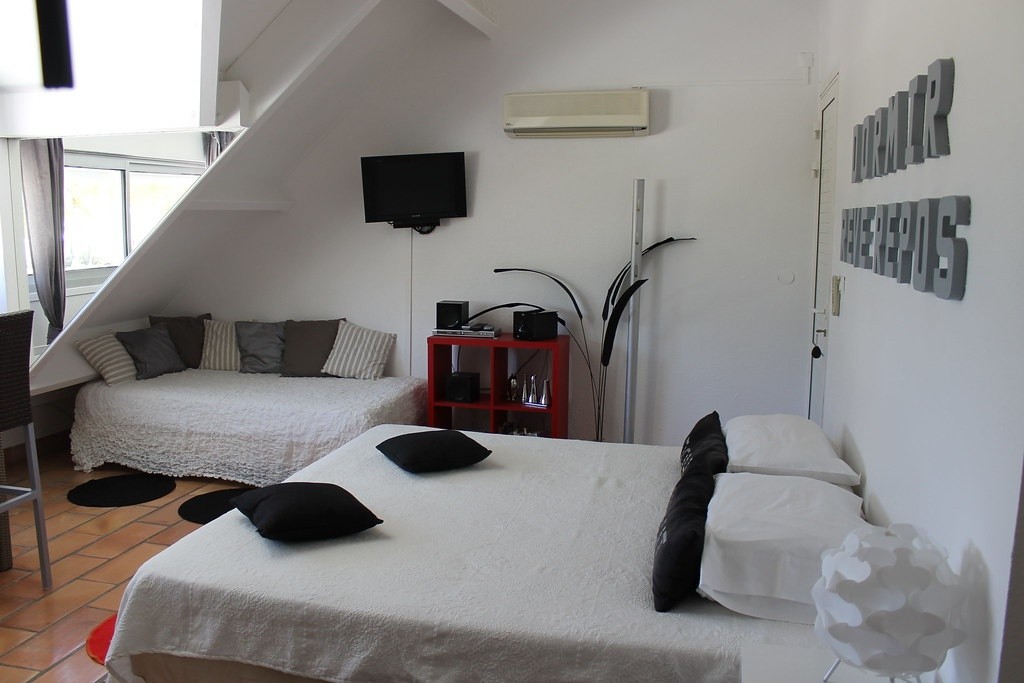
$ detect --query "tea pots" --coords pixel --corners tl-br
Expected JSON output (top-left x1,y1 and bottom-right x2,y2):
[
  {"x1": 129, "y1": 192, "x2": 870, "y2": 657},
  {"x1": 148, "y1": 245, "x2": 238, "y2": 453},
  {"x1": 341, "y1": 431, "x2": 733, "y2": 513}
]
[{"x1": 505, "y1": 374, "x2": 523, "y2": 401}]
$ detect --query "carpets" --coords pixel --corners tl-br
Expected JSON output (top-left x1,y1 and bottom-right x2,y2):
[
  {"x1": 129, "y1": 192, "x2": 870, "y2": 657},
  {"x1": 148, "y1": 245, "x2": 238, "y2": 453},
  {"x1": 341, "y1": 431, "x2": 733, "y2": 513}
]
[
  {"x1": 177, "y1": 490, "x2": 246, "y2": 524},
  {"x1": 67, "y1": 474, "x2": 176, "y2": 509}
]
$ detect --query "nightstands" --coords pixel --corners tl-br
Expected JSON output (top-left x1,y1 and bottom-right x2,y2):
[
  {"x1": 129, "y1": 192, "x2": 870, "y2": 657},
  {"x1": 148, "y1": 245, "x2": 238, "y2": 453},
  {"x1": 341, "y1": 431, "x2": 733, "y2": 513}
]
[{"x1": 739, "y1": 632, "x2": 941, "y2": 683}]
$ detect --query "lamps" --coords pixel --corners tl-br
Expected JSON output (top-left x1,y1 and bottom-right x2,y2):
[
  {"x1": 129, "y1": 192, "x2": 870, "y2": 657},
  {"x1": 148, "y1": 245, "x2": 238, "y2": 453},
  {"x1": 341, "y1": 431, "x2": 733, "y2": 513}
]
[
  {"x1": 812, "y1": 525, "x2": 969, "y2": 682},
  {"x1": 463, "y1": 236, "x2": 695, "y2": 442}
]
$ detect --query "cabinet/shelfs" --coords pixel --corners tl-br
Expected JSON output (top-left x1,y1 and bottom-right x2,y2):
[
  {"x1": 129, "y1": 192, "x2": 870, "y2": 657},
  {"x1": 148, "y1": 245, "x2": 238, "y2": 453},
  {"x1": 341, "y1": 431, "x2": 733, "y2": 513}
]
[{"x1": 427, "y1": 334, "x2": 570, "y2": 440}]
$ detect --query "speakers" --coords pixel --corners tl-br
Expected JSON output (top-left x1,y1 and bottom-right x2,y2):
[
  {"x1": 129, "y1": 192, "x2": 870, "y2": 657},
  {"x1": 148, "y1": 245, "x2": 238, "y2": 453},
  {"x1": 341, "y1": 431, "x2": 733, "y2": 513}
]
[
  {"x1": 513, "y1": 310, "x2": 558, "y2": 341},
  {"x1": 436, "y1": 300, "x2": 468, "y2": 334}
]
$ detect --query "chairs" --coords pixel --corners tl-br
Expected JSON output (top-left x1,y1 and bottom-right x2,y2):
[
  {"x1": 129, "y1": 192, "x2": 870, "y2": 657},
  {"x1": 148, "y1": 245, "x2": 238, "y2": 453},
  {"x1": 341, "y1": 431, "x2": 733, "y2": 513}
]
[{"x1": 0, "y1": 308, "x2": 53, "y2": 591}]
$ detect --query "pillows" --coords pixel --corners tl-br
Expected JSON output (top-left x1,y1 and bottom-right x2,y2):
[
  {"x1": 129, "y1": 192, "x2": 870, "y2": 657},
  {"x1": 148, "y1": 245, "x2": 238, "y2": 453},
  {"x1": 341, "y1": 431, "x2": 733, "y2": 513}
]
[
  {"x1": 75, "y1": 311, "x2": 396, "y2": 387},
  {"x1": 230, "y1": 481, "x2": 384, "y2": 545},
  {"x1": 376, "y1": 429, "x2": 494, "y2": 474},
  {"x1": 649, "y1": 411, "x2": 866, "y2": 624}
]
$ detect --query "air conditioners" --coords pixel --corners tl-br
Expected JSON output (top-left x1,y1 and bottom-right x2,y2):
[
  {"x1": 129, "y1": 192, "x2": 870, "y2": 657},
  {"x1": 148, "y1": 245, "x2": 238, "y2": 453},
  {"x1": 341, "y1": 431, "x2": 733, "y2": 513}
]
[{"x1": 503, "y1": 88, "x2": 650, "y2": 139}]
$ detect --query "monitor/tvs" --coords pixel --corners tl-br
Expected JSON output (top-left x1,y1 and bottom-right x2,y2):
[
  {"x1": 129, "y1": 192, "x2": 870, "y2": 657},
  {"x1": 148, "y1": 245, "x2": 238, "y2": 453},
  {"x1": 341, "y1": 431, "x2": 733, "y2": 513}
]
[{"x1": 360, "y1": 152, "x2": 468, "y2": 223}]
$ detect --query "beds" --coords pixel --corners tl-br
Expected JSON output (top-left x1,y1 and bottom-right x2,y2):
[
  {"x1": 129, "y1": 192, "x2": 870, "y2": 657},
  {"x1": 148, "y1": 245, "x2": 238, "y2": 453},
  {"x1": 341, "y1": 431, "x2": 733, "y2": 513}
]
[
  {"x1": 68, "y1": 369, "x2": 429, "y2": 488},
  {"x1": 106, "y1": 422, "x2": 821, "y2": 683}
]
